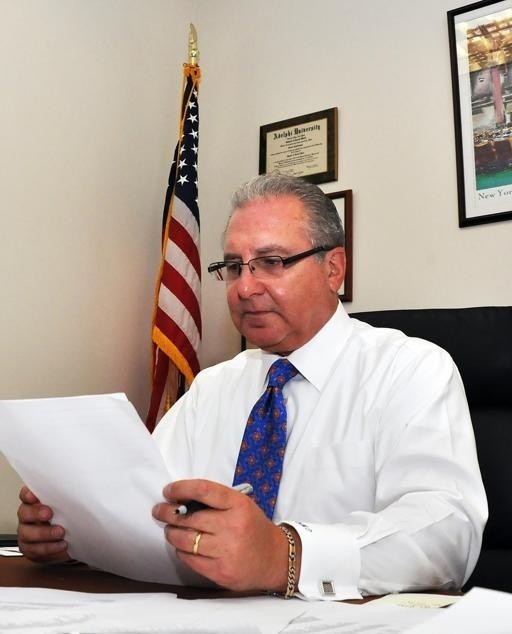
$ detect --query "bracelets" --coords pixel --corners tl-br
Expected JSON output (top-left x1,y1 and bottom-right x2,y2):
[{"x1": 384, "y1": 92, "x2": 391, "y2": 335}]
[{"x1": 276, "y1": 524, "x2": 299, "y2": 600}]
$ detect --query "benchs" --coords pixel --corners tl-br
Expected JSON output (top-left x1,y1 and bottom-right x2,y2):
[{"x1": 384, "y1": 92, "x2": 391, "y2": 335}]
[{"x1": 347, "y1": 306, "x2": 512, "y2": 594}]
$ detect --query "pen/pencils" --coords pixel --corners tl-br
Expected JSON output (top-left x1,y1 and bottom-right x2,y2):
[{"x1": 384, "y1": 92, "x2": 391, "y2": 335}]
[{"x1": 173, "y1": 483, "x2": 253, "y2": 515}]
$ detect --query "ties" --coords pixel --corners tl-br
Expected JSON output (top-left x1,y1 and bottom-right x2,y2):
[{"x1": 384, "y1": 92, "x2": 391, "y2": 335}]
[{"x1": 232, "y1": 358, "x2": 299, "y2": 521}]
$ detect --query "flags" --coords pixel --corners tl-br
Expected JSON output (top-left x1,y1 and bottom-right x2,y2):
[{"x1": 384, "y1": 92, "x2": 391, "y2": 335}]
[{"x1": 147, "y1": 56, "x2": 203, "y2": 417}]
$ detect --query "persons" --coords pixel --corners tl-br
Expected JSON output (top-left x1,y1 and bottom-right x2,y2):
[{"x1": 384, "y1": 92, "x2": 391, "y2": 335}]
[{"x1": 9, "y1": 170, "x2": 491, "y2": 597}]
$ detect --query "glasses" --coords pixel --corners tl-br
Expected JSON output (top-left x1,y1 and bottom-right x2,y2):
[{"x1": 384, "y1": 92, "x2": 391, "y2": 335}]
[{"x1": 207, "y1": 243, "x2": 343, "y2": 281}]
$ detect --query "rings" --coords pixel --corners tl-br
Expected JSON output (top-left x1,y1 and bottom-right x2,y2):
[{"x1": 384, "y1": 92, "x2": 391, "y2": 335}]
[{"x1": 192, "y1": 530, "x2": 204, "y2": 555}]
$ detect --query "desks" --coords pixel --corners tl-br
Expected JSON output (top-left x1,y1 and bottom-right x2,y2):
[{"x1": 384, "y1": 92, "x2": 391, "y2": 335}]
[{"x1": 0, "y1": 544, "x2": 277, "y2": 600}]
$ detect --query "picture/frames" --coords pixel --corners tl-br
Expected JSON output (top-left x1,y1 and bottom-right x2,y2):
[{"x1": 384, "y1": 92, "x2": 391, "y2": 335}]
[
  {"x1": 447, "y1": 0, "x2": 512, "y2": 228},
  {"x1": 326, "y1": 188, "x2": 352, "y2": 302}
]
